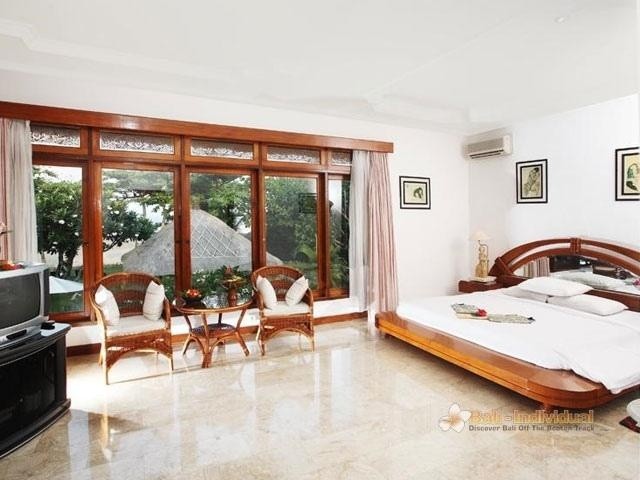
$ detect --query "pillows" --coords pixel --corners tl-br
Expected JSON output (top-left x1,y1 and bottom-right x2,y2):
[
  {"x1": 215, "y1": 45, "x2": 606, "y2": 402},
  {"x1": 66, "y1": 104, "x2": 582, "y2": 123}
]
[
  {"x1": 519, "y1": 277, "x2": 594, "y2": 297},
  {"x1": 95, "y1": 283, "x2": 120, "y2": 326},
  {"x1": 143, "y1": 280, "x2": 164, "y2": 321},
  {"x1": 256, "y1": 275, "x2": 277, "y2": 309},
  {"x1": 548, "y1": 293, "x2": 630, "y2": 316},
  {"x1": 502, "y1": 285, "x2": 549, "y2": 303},
  {"x1": 285, "y1": 275, "x2": 308, "y2": 306}
]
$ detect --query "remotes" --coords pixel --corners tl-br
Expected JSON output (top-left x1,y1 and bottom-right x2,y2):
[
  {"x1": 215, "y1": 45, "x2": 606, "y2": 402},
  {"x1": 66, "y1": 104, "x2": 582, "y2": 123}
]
[{"x1": 43, "y1": 320, "x2": 55, "y2": 324}]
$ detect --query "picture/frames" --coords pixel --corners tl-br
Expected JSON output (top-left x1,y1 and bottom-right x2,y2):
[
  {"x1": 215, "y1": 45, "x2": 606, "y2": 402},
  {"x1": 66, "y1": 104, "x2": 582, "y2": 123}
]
[
  {"x1": 516, "y1": 159, "x2": 548, "y2": 204},
  {"x1": 399, "y1": 175, "x2": 431, "y2": 210},
  {"x1": 615, "y1": 146, "x2": 640, "y2": 201}
]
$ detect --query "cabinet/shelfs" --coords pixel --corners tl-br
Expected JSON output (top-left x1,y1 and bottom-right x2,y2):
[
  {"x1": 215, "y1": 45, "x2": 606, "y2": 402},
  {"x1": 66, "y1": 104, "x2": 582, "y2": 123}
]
[{"x1": 0, "y1": 324, "x2": 72, "y2": 459}]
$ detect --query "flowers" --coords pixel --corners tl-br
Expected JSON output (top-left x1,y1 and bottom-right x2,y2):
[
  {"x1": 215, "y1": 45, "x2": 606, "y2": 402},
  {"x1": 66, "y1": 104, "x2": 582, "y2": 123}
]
[{"x1": 210, "y1": 265, "x2": 252, "y2": 292}]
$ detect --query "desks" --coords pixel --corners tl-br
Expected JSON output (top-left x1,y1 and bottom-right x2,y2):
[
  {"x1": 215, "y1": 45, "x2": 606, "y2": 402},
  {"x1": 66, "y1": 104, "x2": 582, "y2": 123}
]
[{"x1": 174, "y1": 295, "x2": 254, "y2": 368}]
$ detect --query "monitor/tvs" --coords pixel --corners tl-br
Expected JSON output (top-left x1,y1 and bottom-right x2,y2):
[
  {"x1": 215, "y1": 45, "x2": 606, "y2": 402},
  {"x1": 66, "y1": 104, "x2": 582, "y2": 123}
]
[{"x1": 0, "y1": 263, "x2": 49, "y2": 350}]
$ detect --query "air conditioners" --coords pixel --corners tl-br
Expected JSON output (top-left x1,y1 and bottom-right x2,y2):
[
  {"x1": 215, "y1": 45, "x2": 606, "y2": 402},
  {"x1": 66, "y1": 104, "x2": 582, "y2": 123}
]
[{"x1": 465, "y1": 132, "x2": 513, "y2": 160}]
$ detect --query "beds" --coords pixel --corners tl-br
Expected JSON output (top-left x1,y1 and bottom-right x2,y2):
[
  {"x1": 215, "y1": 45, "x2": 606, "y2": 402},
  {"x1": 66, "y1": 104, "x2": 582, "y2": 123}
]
[{"x1": 375, "y1": 238, "x2": 640, "y2": 415}]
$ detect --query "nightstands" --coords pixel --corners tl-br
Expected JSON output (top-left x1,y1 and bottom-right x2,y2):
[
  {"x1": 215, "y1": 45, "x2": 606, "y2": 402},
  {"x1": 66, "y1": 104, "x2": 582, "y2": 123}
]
[{"x1": 459, "y1": 280, "x2": 503, "y2": 293}]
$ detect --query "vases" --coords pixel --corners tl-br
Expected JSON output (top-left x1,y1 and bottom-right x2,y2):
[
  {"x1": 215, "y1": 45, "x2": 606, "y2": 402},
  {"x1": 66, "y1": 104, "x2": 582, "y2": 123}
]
[{"x1": 228, "y1": 289, "x2": 237, "y2": 306}]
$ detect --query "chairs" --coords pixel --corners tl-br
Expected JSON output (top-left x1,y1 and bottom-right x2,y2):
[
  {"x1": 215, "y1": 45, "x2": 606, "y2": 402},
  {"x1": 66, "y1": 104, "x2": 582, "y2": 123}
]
[
  {"x1": 251, "y1": 265, "x2": 315, "y2": 356},
  {"x1": 88, "y1": 272, "x2": 174, "y2": 385}
]
